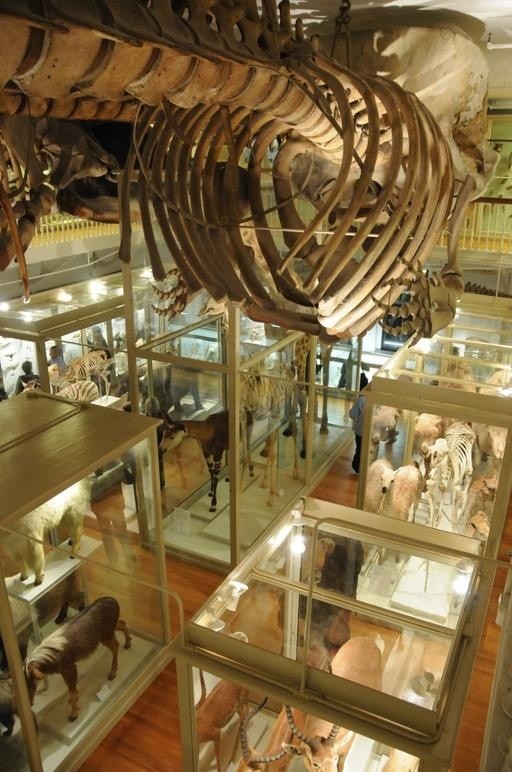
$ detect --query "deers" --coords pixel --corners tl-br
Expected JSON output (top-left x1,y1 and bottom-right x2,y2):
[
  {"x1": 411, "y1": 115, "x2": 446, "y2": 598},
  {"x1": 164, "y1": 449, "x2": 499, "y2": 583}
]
[
  {"x1": 193, "y1": 666, "x2": 250, "y2": 772},
  {"x1": 284, "y1": 636, "x2": 384, "y2": 771},
  {"x1": 237, "y1": 697, "x2": 306, "y2": 772},
  {"x1": 159, "y1": 410, "x2": 254, "y2": 514}
]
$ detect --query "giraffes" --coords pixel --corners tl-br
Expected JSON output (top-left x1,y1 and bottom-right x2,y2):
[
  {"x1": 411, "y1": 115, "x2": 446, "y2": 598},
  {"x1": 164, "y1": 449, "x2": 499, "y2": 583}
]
[{"x1": 239, "y1": 220, "x2": 334, "y2": 459}]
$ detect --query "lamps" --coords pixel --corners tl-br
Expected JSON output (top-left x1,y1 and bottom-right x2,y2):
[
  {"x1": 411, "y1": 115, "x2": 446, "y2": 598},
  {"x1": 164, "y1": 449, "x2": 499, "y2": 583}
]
[
  {"x1": 217, "y1": 581, "x2": 249, "y2": 612},
  {"x1": 410, "y1": 671, "x2": 437, "y2": 698},
  {"x1": 226, "y1": 631, "x2": 248, "y2": 644},
  {"x1": 455, "y1": 558, "x2": 472, "y2": 574},
  {"x1": 205, "y1": 607, "x2": 225, "y2": 632}
]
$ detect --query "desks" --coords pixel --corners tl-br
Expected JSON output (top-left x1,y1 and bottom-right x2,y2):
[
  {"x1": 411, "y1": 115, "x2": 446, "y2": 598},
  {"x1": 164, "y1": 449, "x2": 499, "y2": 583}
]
[{"x1": 6, "y1": 534, "x2": 104, "y2": 646}]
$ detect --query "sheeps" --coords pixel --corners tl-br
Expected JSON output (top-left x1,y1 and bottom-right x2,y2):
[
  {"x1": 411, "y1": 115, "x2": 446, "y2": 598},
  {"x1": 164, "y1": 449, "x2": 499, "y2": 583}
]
[
  {"x1": 471, "y1": 369, "x2": 511, "y2": 465},
  {"x1": 412, "y1": 369, "x2": 479, "y2": 493},
  {"x1": 1, "y1": 596, "x2": 132, "y2": 721}
]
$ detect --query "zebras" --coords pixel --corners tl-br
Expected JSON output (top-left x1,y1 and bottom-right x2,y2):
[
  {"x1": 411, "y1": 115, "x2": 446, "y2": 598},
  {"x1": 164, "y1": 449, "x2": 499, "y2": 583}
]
[{"x1": 55, "y1": 379, "x2": 99, "y2": 403}]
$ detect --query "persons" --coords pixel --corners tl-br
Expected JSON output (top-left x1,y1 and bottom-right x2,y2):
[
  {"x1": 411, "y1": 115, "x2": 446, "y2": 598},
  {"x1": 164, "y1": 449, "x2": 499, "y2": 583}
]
[
  {"x1": 338, "y1": 349, "x2": 370, "y2": 423},
  {"x1": 90, "y1": 461, "x2": 138, "y2": 565},
  {"x1": 47, "y1": 345, "x2": 67, "y2": 371},
  {"x1": 86, "y1": 325, "x2": 112, "y2": 360},
  {"x1": 14, "y1": 361, "x2": 40, "y2": 396},
  {"x1": 348, "y1": 396, "x2": 379, "y2": 476},
  {"x1": 119, "y1": 400, "x2": 139, "y2": 515},
  {"x1": 145, "y1": 396, "x2": 170, "y2": 513},
  {"x1": 297, "y1": 538, "x2": 363, "y2": 622}
]
